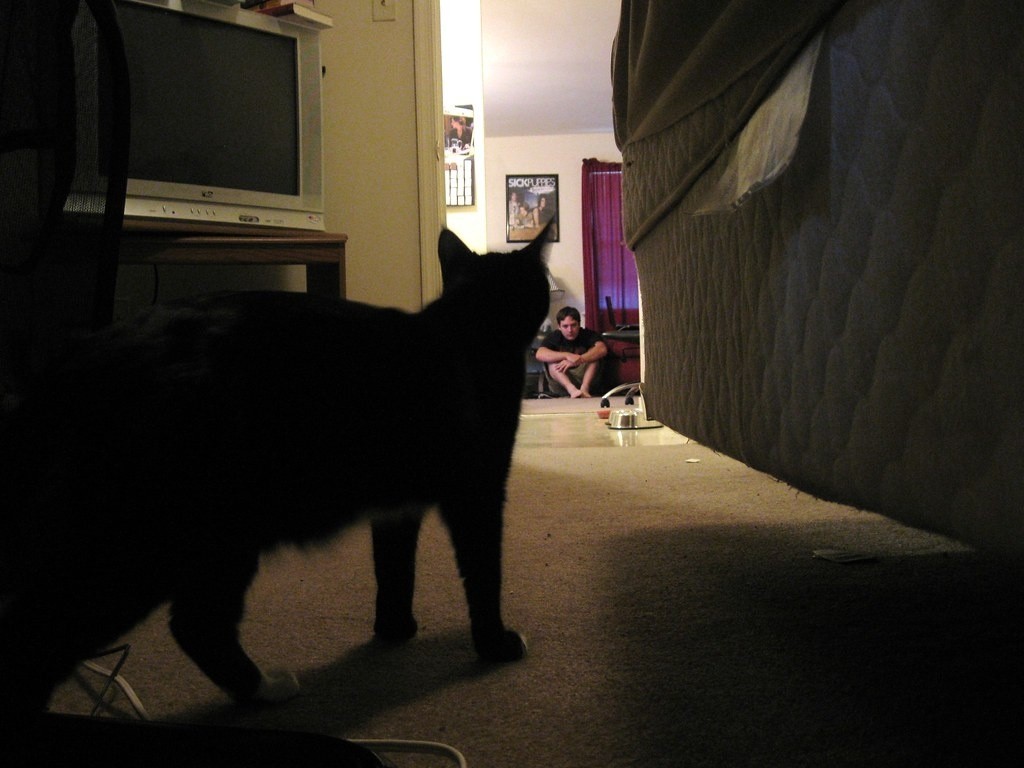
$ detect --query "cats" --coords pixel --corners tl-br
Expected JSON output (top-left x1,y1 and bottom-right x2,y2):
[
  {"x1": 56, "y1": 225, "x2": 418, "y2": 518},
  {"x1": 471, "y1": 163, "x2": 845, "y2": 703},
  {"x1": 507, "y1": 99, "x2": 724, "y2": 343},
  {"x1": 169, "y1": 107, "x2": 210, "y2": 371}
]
[{"x1": 0, "y1": 215, "x2": 560, "y2": 741}]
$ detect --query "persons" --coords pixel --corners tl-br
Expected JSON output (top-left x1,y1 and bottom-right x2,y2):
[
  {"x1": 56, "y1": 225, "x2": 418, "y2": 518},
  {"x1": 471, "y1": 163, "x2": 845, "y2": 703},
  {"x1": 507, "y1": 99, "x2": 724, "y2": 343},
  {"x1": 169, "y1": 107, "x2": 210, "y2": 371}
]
[
  {"x1": 508, "y1": 191, "x2": 553, "y2": 228},
  {"x1": 535, "y1": 306, "x2": 609, "y2": 399},
  {"x1": 448, "y1": 116, "x2": 474, "y2": 148}
]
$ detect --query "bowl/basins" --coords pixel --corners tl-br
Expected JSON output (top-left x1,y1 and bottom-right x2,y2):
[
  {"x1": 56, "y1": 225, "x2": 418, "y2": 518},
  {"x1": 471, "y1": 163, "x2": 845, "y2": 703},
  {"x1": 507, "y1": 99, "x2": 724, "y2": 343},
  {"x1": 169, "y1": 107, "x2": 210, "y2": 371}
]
[
  {"x1": 609, "y1": 413, "x2": 638, "y2": 429},
  {"x1": 609, "y1": 410, "x2": 634, "y2": 422},
  {"x1": 596, "y1": 408, "x2": 611, "y2": 418}
]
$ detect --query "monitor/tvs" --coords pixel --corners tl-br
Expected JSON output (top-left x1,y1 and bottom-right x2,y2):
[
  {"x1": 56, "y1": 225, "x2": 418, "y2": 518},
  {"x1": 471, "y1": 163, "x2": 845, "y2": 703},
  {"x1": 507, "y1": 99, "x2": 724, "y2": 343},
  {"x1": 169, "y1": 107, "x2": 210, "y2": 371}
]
[{"x1": 60, "y1": 0, "x2": 324, "y2": 231}]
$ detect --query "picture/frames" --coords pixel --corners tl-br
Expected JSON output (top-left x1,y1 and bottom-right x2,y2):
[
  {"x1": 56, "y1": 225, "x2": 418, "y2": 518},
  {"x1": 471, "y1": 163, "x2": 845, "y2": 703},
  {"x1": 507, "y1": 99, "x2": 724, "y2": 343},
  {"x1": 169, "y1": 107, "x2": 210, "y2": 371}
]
[{"x1": 505, "y1": 174, "x2": 560, "y2": 243}]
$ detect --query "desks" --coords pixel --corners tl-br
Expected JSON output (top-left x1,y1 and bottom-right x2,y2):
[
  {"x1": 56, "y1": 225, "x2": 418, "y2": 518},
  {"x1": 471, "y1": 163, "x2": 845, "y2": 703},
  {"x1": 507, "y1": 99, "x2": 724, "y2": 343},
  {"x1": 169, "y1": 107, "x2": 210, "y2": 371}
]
[{"x1": 0, "y1": 231, "x2": 348, "y2": 331}]
